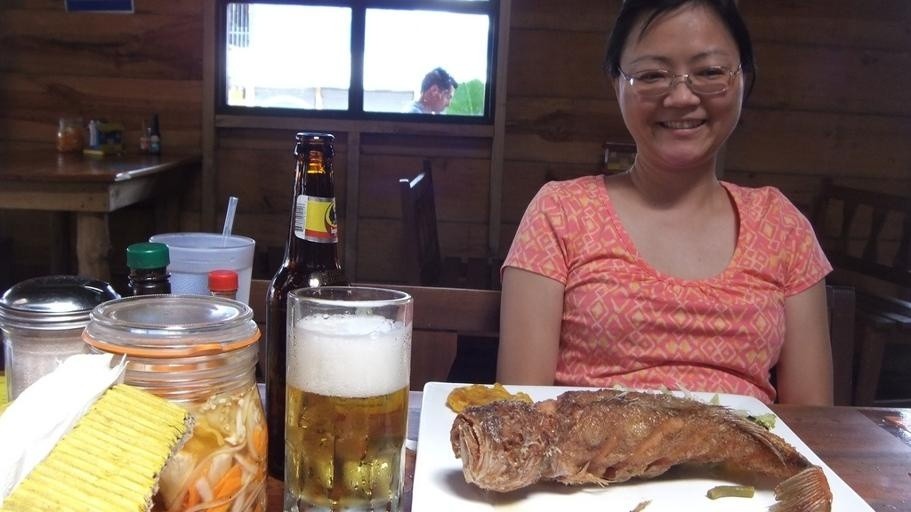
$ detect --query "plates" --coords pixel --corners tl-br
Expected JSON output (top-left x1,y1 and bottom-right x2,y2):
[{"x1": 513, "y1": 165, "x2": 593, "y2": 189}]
[{"x1": 410, "y1": 377, "x2": 879, "y2": 512}]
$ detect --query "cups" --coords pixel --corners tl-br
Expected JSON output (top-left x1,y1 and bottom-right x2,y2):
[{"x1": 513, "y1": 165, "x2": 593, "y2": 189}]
[
  {"x1": 150, "y1": 231, "x2": 255, "y2": 303},
  {"x1": 283, "y1": 282, "x2": 416, "y2": 512}
]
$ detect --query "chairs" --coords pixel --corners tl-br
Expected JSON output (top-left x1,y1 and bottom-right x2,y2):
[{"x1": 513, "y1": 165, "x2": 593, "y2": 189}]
[{"x1": 399, "y1": 158, "x2": 505, "y2": 291}]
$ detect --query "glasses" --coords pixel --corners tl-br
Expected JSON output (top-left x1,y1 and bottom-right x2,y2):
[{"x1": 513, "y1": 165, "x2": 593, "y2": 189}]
[{"x1": 617, "y1": 63, "x2": 742, "y2": 98}]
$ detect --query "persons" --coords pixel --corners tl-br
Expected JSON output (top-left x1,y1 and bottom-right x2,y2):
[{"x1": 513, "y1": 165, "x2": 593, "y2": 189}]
[
  {"x1": 411, "y1": 68, "x2": 460, "y2": 117},
  {"x1": 493, "y1": 1, "x2": 841, "y2": 410}
]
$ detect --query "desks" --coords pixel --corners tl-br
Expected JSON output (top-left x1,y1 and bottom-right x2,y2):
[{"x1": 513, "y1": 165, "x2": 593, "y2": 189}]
[{"x1": 0, "y1": 148, "x2": 184, "y2": 280}]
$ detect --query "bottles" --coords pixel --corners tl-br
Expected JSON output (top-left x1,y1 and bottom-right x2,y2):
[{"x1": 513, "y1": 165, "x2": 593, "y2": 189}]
[
  {"x1": 86, "y1": 293, "x2": 270, "y2": 512},
  {"x1": 264, "y1": 130, "x2": 352, "y2": 484},
  {"x1": 1, "y1": 273, "x2": 120, "y2": 401},
  {"x1": 140, "y1": 112, "x2": 162, "y2": 158},
  {"x1": 56, "y1": 116, "x2": 85, "y2": 153},
  {"x1": 124, "y1": 242, "x2": 170, "y2": 298}
]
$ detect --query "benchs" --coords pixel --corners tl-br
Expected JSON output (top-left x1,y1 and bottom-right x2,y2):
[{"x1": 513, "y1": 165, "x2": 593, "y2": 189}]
[
  {"x1": 250, "y1": 280, "x2": 856, "y2": 405},
  {"x1": 804, "y1": 180, "x2": 910, "y2": 323}
]
